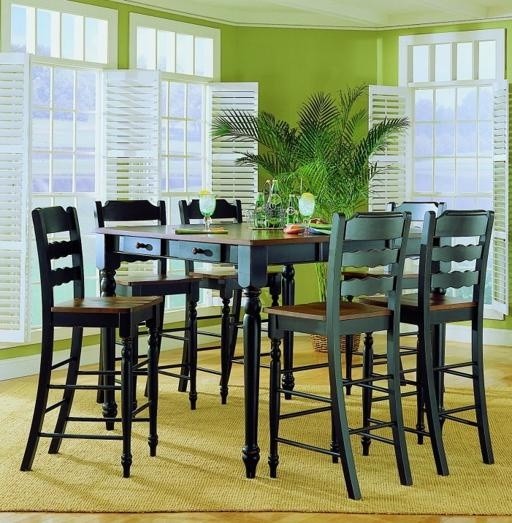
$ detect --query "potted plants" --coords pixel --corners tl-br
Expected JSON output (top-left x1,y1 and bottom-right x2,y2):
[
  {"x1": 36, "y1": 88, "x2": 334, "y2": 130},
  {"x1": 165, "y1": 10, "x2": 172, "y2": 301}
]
[{"x1": 209, "y1": 78, "x2": 413, "y2": 354}]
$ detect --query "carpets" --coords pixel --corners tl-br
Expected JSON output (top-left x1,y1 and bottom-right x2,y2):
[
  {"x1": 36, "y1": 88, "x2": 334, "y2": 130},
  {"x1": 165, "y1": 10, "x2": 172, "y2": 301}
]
[{"x1": 1, "y1": 333, "x2": 512, "y2": 515}]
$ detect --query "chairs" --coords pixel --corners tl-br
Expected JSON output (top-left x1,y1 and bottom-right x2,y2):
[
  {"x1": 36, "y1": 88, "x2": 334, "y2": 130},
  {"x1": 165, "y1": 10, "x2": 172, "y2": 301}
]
[
  {"x1": 262, "y1": 212, "x2": 413, "y2": 501},
  {"x1": 357, "y1": 208, "x2": 496, "y2": 476},
  {"x1": 19, "y1": 205, "x2": 164, "y2": 479},
  {"x1": 94, "y1": 199, "x2": 200, "y2": 411},
  {"x1": 344, "y1": 200, "x2": 448, "y2": 396},
  {"x1": 178, "y1": 199, "x2": 294, "y2": 404}
]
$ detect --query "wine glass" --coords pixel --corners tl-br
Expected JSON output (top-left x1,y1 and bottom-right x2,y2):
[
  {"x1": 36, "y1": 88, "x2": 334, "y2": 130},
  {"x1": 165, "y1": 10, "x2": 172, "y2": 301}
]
[
  {"x1": 297, "y1": 194, "x2": 316, "y2": 236},
  {"x1": 198, "y1": 195, "x2": 216, "y2": 231}
]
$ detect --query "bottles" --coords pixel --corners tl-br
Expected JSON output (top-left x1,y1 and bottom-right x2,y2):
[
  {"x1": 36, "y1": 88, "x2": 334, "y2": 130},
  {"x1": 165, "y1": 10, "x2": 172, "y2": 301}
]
[
  {"x1": 268, "y1": 180, "x2": 282, "y2": 227},
  {"x1": 286, "y1": 194, "x2": 296, "y2": 224},
  {"x1": 254, "y1": 193, "x2": 266, "y2": 229}
]
